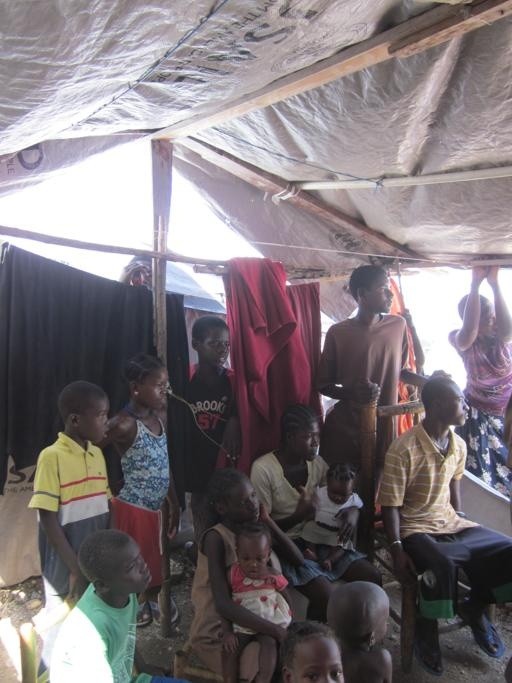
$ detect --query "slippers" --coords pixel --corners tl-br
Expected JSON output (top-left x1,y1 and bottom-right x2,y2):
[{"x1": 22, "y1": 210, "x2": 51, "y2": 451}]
[
  {"x1": 136, "y1": 601, "x2": 150, "y2": 626},
  {"x1": 148, "y1": 596, "x2": 178, "y2": 625}
]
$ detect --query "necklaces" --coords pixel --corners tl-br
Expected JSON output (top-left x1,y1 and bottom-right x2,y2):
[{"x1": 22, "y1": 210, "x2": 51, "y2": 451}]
[{"x1": 433, "y1": 438, "x2": 450, "y2": 452}]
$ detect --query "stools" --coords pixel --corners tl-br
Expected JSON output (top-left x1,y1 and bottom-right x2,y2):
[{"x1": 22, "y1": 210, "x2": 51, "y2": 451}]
[{"x1": 174, "y1": 643, "x2": 224, "y2": 682}]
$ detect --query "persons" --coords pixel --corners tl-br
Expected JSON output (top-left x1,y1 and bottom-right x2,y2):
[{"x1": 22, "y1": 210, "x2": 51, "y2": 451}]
[
  {"x1": 187, "y1": 467, "x2": 306, "y2": 682},
  {"x1": 304, "y1": 465, "x2": 368, "y2": 573},
  {"x1": 251, "y1": 403, "x2": 382, "y2": 620},
  {"x1": 278, "y1": 619, "x2": 344, "y2": 683},
  {"x1": 25, "y1": 380, "x2": 113, "y2": 683},
  {"x1": 376, "y1": 376, "x2": 511, "y2": 678},
  {"x1": 93, "y1": 348, "x2": 180, "y2": 628},
  {"x1": 49, "y1": 529, "x2": 190, "y2": 683},
  {"x1": 447, "y1": 263, "x2": 511, "y2": 501},
  {"x1": 221, "y1": 520, "x2": 293, "y2": 683},
  {"x1": 325, "y1": 581, "x2": 394, "y2": 683},
  {"x1": 314, "y1": 264, "x2": 412, "y2": 493},
  {"x1": 167, "y1": 317, "x2": 242, "y2": 546}
]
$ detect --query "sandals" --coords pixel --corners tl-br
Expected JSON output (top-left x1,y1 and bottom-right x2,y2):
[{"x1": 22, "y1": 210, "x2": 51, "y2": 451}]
[
  {"x1": 415, "y1": 619, "x2": 443, "y2": 675},
  {"x1": 457, "y1": 602, "x2": 503, "y2": 658}
]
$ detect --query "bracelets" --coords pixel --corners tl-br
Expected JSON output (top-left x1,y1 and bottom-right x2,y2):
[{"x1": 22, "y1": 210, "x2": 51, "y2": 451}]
[{"x1": 389, "y1": 540, "x2": 403, "y2": 549}]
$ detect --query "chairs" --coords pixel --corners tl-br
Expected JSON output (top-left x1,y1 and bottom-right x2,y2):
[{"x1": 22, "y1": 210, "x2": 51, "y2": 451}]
[
  {"x1": 20, "y1": 592, "x2": 78, "y2": 683},
  {"x1": 368, "y1": 400, "x2": 496, "y2": 674}
]
